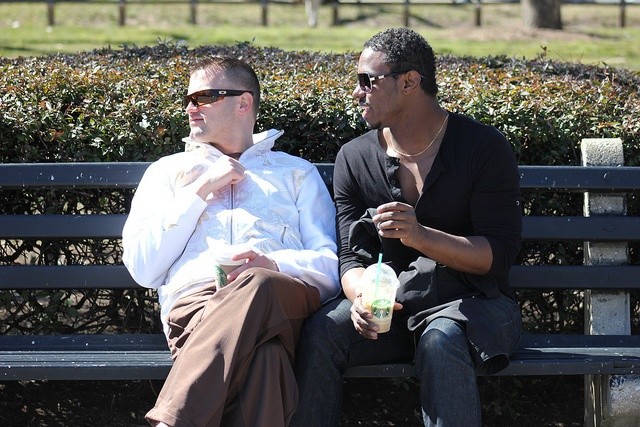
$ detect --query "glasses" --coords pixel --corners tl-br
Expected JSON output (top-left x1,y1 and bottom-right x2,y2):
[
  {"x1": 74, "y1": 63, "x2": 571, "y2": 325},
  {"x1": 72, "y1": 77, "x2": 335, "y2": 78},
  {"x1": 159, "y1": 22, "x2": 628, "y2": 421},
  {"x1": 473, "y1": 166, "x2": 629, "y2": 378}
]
[
  {"x1": 356, "y1": 70, "x2": 427, "y2": 93},
  {"x1": 183, "y1": 89, "x2": 254, "y2": 109}
]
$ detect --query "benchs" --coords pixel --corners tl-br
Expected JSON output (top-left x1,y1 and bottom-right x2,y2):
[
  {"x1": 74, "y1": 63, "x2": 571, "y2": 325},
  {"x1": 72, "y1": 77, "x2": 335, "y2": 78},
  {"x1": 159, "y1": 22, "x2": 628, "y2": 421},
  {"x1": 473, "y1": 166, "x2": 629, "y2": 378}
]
[{"x1": 1, "y1": 138, "x2": 639, "y2": 425}]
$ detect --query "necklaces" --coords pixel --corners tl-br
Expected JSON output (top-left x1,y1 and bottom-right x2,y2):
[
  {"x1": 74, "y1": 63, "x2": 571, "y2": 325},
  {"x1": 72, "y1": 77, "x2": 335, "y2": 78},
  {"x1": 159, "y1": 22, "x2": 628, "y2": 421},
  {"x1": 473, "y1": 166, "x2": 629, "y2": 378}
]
[{"x1": 389, "y1": 114, "x2": 449, "y2": 157}]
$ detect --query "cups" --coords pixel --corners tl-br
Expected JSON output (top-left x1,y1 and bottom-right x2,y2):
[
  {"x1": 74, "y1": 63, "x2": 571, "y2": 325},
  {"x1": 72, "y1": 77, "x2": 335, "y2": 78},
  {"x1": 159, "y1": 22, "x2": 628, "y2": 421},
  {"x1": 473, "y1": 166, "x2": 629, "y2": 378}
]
[
  {"x1": 356, "y1": 264, "x2": 401, "y2": 334},
  {"x1": 214, "y1": 248, "x2": 246, "y2": 289}
]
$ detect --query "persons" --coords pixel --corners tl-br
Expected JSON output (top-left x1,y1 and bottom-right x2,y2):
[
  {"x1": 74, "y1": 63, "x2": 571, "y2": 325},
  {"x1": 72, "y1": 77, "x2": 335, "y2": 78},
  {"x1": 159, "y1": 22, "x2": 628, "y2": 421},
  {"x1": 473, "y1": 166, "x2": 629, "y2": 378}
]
[
  {"x1": 295, "y1": 27, "x2": 522, "y2": 427},
  {"x1": 121, "y1": 56, "x2": 342, "y2": 426}
]
